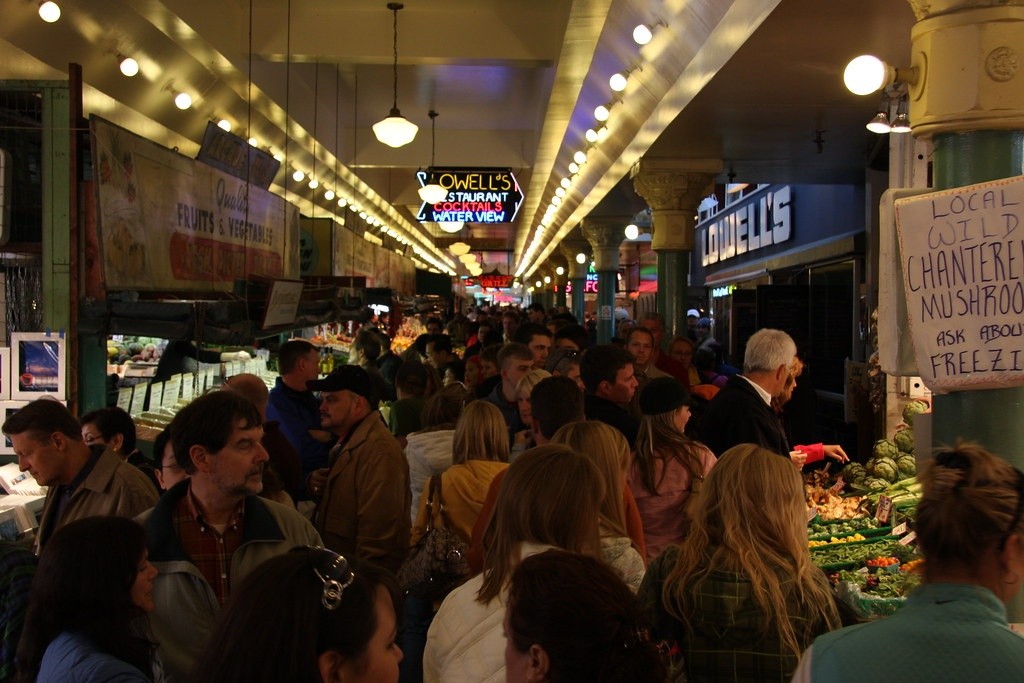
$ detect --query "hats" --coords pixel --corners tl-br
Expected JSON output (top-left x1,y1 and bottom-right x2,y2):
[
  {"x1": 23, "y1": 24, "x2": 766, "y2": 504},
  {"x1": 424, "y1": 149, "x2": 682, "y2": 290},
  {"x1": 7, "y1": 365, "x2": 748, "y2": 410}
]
[
  {"x1": 640, "y1": 377, "x2": 698, "y2": 415},
  {"x1": 305, "y1": 365, "x2": 371, "y2": 397},
  {"x1": 687, "y1": 308, "x2": 700, "y2": 317},
  {"x1": 697, "y1": 318, "x2": 710, "y2": 327}
]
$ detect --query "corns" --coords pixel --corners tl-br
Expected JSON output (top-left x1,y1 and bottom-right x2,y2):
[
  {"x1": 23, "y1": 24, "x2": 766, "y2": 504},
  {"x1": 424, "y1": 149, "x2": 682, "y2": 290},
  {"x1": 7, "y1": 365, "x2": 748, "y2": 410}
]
[{"x1": 868, "y1": 478, "x2": 923, "y2": 509}]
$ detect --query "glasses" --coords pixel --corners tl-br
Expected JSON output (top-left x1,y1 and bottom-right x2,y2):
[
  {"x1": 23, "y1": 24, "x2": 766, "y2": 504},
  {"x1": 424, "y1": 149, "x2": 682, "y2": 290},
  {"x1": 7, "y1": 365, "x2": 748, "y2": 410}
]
[
  {"x1": 996, "y1": 465, "x2": 1024, "y2": 552},
  {"x1": 551, "y1": 351, "x2": 578, "y2": 371},
  {"x1": 83, "y1": 433, "x2": 108, "y2": 446},
  {"x1": 290, "y1": 544, "x2": 354, "y2": 651}
]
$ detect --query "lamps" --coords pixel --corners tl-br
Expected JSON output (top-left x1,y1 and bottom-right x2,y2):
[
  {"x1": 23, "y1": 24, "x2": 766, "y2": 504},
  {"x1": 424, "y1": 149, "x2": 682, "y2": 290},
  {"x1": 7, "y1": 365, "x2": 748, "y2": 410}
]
[
  {"x1": 839, "y1": 55, "x2": 921, "y2": 136},
  {"x1": 32, "y1": 1, "x2": 487, "y2": 284},
  {"x1": 518, "y1": 17, "x2": 667, "y2": 292},
  {"x1": 698, "y1": 195, "x2": 718, "y2": 213},
  {"x1": 727, "y1": 183, "x2": 747, "y2": 193}
]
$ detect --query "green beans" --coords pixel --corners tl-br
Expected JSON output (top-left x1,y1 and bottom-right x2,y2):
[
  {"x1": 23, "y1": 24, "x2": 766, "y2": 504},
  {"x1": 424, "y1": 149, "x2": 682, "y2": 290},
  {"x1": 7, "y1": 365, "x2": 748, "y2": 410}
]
[{"x1": 811, "y1": 538, "x2": 915, "y2": 566}]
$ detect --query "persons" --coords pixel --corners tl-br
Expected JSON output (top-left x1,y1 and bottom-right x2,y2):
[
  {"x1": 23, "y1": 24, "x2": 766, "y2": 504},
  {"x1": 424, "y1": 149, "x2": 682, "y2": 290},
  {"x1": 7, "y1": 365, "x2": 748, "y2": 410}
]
[
  {"x1": 142, "y1": 392, "x2": 329, "y2": 616},
  {"x1": 0, "y1": 538, "x2": 39, "y2": 683},
  {"x1": 36, "y1": 517, "x2": 165, "y2": 683},
  {"x1": 224, "y1": 302, "x2": 804, "y2": 537},
  {"x1": 691, "y1": 329, "x2": 797, "y2": 460},
  {"x1": 505, "y1": 550, "x2": 663, "y2": 683},
  {"x1": 3, "y1": 399, "x2": 160, "y2": 558},
  {"x1": 308, "y1": 365, "x2": 412, "y2": 584},
  {"x1": 410, "y1": 400, "x2": 512, "y2": 598},
  {"x1": 423, "y1": 443, "x2": 636, "y2": 683},
  {"x1": 635, "y1": 444, "x2": 861, "y2": 683},
  {"x1": 791, "y1": 439, "x2": 1024, "y2": 683},
  {"x1": 81, "y1": 408, "x2": 161, "y2": 497},
  {"x1": 188, "y1": 544, "x2": 403, "y2": 683}
]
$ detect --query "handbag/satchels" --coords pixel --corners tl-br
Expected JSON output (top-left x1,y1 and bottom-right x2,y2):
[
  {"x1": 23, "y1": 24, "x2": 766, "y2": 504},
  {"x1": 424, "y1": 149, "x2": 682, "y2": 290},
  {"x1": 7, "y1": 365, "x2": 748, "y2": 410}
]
[{"x1": 394, "y1": 472, "x2": 469, "y2": 617}]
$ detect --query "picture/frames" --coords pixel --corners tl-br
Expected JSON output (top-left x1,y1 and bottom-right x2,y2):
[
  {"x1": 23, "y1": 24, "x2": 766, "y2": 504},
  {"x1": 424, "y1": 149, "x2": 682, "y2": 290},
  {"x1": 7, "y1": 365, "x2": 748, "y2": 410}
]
[
  {"x1": 0, "y1": 347, "x2": 10, "y2": 401},
  {"x1": 0, "y1": 401, "x2": 68, "y2": 455},
  {"x1": 10, "y1": 332, "x2": 66, "y2": 400}
]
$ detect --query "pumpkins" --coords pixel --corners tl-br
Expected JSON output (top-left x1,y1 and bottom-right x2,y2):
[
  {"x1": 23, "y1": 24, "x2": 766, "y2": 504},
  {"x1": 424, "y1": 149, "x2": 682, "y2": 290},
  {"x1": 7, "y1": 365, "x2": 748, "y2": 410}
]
[{"x1": 107, "y1": 340, "x2": 160, "y2": 365}]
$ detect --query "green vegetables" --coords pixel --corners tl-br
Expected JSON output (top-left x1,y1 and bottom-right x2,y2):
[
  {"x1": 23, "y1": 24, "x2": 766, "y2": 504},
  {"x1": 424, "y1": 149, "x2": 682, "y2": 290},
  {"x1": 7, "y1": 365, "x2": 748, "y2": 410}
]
[{"x1": 831, "y1": 570, "x2": 921, "y2": 597}]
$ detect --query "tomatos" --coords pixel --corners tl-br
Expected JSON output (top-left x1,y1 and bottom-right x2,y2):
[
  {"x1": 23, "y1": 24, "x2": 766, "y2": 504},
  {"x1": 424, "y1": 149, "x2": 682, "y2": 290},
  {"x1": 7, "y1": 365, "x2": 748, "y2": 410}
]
[{"x1": 868, "y1": 555, "x2": 923, "y2": 571}]
